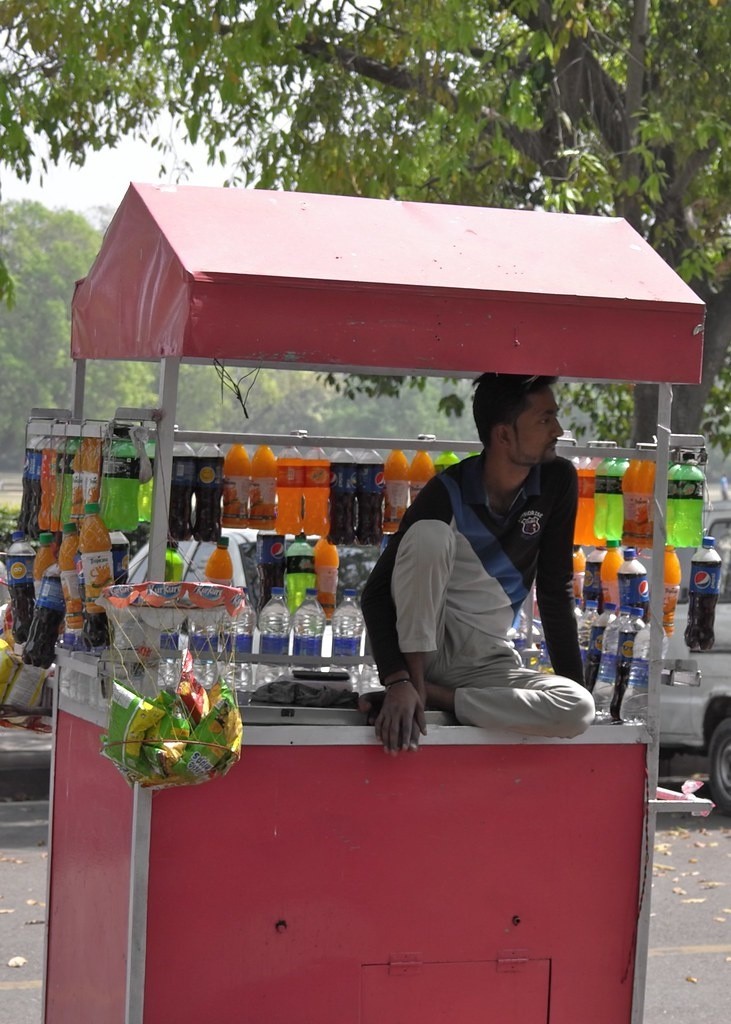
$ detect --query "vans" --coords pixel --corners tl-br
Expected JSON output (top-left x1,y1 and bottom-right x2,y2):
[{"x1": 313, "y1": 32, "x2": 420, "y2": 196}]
[{"x1": 595, "y1": 499, "x2": 731, "y2": 815}]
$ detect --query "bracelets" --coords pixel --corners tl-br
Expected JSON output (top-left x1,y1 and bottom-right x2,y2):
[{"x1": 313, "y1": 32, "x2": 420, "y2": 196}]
[{"x1": 385, "y1": 678, "x2": 416, "y2": 689}]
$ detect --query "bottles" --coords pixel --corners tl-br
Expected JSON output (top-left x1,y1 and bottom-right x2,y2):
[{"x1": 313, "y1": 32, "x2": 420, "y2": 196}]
[{"x1": 7, "y1": 426, "x2": 720, "y2": 724}]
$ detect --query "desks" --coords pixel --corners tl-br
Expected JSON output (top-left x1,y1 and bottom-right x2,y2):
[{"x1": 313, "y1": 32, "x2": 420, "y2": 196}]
[{"x1": 41, "y1": 646, "x2": 658, "y2": 1024}]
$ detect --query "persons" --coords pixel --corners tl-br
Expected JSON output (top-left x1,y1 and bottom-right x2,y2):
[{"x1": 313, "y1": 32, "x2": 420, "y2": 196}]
[{"x1": 356, "y1": 372, "x2": 598, "y2": 759}]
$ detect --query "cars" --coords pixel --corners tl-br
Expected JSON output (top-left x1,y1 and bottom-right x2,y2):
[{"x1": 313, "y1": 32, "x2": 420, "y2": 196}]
[{"x1": 80, "y1": 528, "x2": 377, "y2": 679}]
